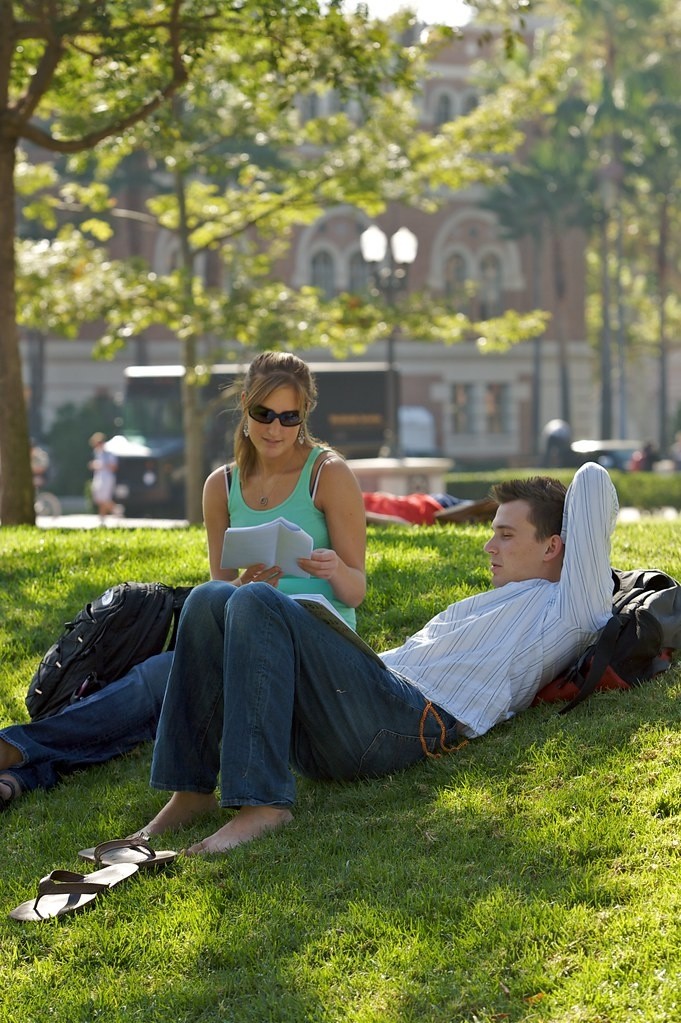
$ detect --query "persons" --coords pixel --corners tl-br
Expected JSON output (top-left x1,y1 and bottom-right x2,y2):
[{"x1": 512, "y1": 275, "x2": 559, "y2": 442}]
[
  {"x1": 670, "y1": 430, "x2": 681, "y2": 473},
  {"x1": 631, "y1": 441, "x2": 660, "y2": 472},
  {"x1": 30, "y1": 436, "x2": 50, "y2": 487},
  {"x1": 127, "y1": 461, "x2": 620, "y2": 857},
  {"x1": 88, "y1": 431, "x2": 119, "y2": 514},
  {"x1": 0, "y1": 349, "x2": 367, "y2": 805}
]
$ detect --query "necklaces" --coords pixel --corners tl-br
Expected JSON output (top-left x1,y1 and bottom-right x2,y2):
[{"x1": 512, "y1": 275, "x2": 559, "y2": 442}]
[{"x1": 255, "y1": 461, "x2": 287, "y2": 506}]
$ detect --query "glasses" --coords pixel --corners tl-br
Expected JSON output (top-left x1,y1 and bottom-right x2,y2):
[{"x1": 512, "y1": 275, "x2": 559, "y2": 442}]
[{"x1": 248, "y1": 403, "x2": 302, "y2": 427}]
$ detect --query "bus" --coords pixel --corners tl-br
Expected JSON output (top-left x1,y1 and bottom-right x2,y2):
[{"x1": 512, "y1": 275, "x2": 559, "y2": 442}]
[{"x1": 121, "y1": 362, "x2": 401, "y2": 519}]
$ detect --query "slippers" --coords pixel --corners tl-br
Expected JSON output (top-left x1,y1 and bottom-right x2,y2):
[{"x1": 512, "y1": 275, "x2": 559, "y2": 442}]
[
  {"x1": 79, "y1": 836, "x2": 177, "y2": 869},
  {"x1": 8, "y1": 862, "x2": 139, "y2": 922}
]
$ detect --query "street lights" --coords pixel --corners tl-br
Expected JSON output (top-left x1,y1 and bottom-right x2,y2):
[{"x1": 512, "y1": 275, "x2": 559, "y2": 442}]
[{"x1": 359, "y1": 224, "x2": 418, "y2": 458}]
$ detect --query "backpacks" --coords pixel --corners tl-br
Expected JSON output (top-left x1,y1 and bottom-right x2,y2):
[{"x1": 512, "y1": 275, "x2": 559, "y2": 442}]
[
  {"x1": 26, "y1": 580, "x2": 192, "y2": 720},
  {"x1": 527, "y1": 571, "x2": 681, "y2": 714}
]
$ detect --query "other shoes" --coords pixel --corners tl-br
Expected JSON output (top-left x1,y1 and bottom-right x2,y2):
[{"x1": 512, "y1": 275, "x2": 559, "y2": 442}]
[{"x1": 0, "y1": 779, "x2": 15, "y2": 811}]
